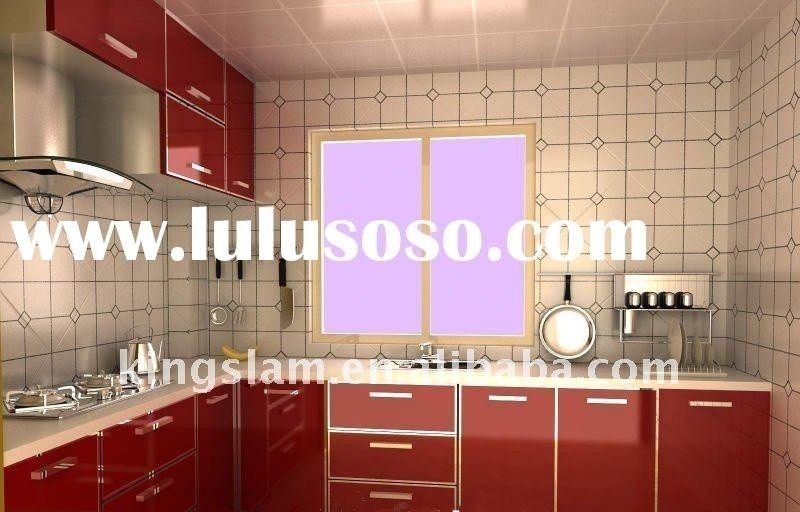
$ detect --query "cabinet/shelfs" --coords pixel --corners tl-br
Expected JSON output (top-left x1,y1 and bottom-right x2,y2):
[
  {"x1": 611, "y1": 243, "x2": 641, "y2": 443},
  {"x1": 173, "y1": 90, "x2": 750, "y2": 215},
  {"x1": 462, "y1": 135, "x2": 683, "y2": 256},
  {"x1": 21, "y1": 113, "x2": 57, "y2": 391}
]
[
  {"x1": 196, "y1": 382, "x2": 235, "y2": 510},
  {"x1": 236, "y1": 376, "x2": 324, "y2": 509},
  {"x1": 45, "y1": 0, "x2": 165, "y2": 94},
  {"x1": 3, "y1": 421, "x2": 101, "y2": 510},
  {"x1": 74, "y1": 8, "x2": 230, "y2": 193},
  {"x1": 226, "y1": 57, "x2": 257, "y2": 202},
  {"x1": 657, "y1": 381, "x2": 773, "y2": 512},
  {"x1": 101, "y1": 388, "x2": 198, "y2": 510},
  {"x1": 325, "y1": 374, "x2": 457, "y2": 511},
  {"x1": 456, "y1": 375, "x2": 657, "y2": 510}
]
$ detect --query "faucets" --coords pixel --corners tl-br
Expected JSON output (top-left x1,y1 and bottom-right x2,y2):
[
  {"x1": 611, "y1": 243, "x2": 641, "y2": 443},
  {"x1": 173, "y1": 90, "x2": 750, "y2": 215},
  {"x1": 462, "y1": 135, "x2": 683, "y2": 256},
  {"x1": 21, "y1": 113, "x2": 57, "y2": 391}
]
[{"x1": 418, "y1": 340, "x2": 434, "y2": 358}]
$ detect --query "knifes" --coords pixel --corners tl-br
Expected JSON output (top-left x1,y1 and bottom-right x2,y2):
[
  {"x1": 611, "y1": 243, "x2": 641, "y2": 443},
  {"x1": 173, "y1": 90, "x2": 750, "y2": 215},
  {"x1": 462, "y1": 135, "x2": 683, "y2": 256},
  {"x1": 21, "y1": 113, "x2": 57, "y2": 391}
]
[{"x1": 279, "y1": 259, "x2": 295, "y2": 331}]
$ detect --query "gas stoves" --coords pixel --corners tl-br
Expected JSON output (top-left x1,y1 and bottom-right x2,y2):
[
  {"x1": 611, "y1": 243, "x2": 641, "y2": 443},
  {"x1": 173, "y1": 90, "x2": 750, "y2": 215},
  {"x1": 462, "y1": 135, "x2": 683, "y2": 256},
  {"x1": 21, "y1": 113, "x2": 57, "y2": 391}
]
[{"x1": 3, "y1": 368, "x2": 177, "y2": 421}]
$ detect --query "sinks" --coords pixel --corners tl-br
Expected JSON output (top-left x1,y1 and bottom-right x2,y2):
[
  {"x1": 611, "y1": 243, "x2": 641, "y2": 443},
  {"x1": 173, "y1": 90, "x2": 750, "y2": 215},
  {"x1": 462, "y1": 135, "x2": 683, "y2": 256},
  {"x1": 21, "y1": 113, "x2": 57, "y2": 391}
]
[{"x1": 405, "y1": 360, "x2": 485, "y2": 370}]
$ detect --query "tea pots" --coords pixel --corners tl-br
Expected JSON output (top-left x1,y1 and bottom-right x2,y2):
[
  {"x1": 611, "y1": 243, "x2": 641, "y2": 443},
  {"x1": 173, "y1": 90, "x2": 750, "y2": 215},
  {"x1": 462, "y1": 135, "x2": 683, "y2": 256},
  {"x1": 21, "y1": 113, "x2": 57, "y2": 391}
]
[{"x1": 124, "y1": 325, "x2": 166, "y2": 375}]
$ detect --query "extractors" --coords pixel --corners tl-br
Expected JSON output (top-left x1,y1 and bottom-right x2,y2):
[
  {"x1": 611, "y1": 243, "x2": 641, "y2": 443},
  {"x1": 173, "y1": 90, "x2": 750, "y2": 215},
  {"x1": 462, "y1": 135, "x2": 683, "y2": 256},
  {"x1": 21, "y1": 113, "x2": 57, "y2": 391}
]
[{"x1": 0, "y1": 31, "x2": 161, "y2": 218}]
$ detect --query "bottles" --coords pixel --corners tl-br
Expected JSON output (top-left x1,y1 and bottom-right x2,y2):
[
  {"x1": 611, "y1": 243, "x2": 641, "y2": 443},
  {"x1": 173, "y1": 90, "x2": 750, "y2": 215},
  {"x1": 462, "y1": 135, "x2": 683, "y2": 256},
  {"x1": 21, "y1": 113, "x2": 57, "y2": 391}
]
[{"x1": 625, "y1": 291, "x2": 694, "y2": 309}]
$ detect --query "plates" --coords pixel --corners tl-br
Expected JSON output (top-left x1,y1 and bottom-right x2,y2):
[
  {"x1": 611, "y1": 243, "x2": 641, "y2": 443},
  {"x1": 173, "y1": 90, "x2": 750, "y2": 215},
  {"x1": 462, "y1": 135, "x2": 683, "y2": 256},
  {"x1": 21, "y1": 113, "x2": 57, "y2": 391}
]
[
  {"x1": 678, "y1": 320, "x2": 688, "y2": 367},
  {"x1": 692, "y1": 336, "x2": 702, "y2": 367},
  {"x1": 667, "y1": 318, "x2": 683, "y2": 365},
  {"x1": 704, "y1": 342, "x2": 713, "y2": 368}
]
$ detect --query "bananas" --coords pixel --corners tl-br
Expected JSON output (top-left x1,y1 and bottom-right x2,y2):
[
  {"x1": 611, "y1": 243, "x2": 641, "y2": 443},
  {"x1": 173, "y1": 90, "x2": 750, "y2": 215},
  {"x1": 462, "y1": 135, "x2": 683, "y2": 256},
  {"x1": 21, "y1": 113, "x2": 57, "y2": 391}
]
[{"x1": 221, "y1": 345, "x2": 259, "y2": 361}]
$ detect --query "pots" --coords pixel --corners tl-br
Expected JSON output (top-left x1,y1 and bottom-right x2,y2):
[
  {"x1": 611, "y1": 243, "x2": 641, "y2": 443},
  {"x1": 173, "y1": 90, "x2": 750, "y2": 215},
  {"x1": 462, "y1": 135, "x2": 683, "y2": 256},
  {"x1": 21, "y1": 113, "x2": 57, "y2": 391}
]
[{"x1": 540, "y1": 275, "x2": 596, "y2": 359}]
